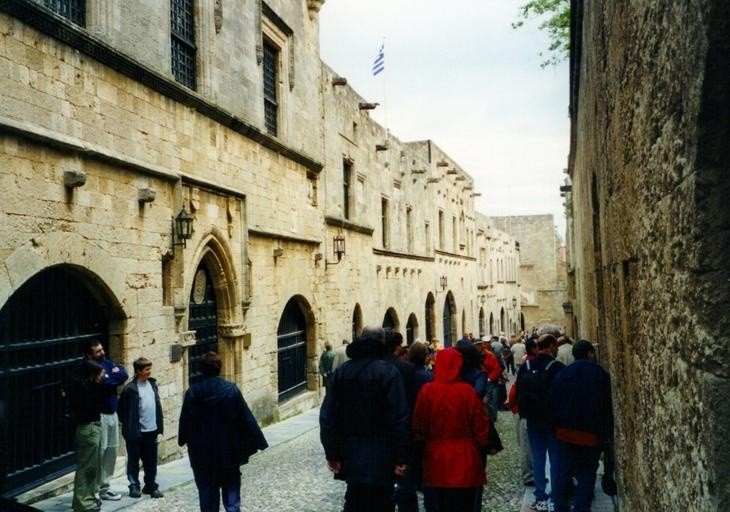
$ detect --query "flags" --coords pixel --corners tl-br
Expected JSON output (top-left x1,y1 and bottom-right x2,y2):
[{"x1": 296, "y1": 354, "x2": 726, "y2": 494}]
[{"x1": 372, "y1": 43, "x2": 385, "y2": 76}]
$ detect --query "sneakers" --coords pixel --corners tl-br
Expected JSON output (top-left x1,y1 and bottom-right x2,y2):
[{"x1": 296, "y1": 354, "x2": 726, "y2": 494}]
[
  {"x1": 522, "y1": 472, "x2": 596, "y2": 512},
  {"x1": 90, "y1": 483, "x2": 164, "y2": 511}
]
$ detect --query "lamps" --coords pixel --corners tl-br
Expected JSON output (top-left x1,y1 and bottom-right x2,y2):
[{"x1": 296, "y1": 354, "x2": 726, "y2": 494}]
[
  {"x1": 325, "y1": 227, "x2": 345, "y2": 271},
  {"x1": 172, "y1": 203, "x2": 193, "y2": 249}
]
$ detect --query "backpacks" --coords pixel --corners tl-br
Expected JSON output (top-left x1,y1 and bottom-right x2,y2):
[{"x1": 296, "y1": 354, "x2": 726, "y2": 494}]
[{"x1": 517, "y1": 359, "x2": 560, "y2": 419}]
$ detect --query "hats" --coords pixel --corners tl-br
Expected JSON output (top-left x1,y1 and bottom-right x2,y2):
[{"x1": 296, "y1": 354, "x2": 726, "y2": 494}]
[
  {"x1": 473, "y1": 335, "x2": 491, "y2": 345},
  {"x1": 572, "y1": 340, "x2": 600, "y2": 357}
]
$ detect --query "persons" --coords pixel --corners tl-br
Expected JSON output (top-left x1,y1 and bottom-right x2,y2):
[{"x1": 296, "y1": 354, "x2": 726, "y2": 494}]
[
  {"x1": 316, "y1": 322, "x2": 613, "y2": 512},
  {"x1": 68, "y1": 357, "x2": 109, "y2": 512},
  {"x1": 86, "y1": 340, "x2": 130, "y2": 505},
  {"x1": 178, "y1": 350, "x2": 269, "y2": 511},
  {"x1": 117, "y1": 355, "x2": 165, "y2": 498}
]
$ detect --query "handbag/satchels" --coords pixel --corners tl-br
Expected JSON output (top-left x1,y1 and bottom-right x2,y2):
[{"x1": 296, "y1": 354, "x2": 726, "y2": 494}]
[{"x1": 601, "y1": 474, "x2": 617, "y2": 495}]
